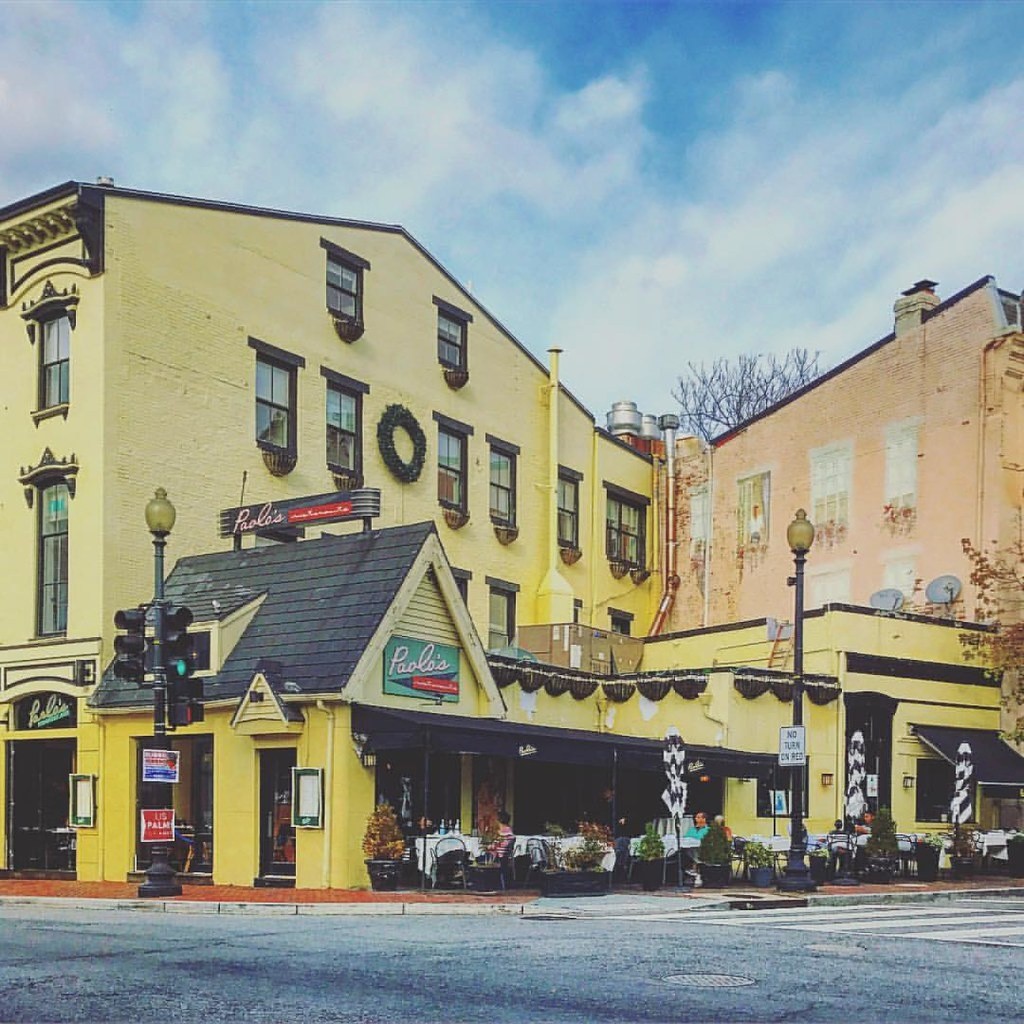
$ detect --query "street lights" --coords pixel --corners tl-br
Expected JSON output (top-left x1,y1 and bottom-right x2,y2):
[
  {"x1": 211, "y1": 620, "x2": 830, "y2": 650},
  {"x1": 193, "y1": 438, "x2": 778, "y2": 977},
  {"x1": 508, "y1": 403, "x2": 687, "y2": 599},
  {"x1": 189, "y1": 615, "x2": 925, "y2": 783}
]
[
  {"x1": 135, "y1": 487, "x2": 177, "y2": 898},
  {"x1": 774, "y1": 506, "x2": 820, "y2": 890}
]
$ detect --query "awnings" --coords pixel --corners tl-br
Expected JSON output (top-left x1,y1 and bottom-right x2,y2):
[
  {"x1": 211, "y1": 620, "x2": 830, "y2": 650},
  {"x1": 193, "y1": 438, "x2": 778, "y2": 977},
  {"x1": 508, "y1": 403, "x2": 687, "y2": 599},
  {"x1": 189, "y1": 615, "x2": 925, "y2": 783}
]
[{"x1": 912, "y1": 724, "x2": 1024, "y2": 788}]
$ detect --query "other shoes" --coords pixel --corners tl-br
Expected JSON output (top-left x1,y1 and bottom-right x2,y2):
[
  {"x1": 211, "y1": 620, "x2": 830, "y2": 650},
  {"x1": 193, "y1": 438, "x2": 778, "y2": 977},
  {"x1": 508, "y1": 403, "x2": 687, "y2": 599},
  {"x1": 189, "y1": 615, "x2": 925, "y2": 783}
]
[{"x1": 685, "y1": 869, "x2": 698, "y2": 876}]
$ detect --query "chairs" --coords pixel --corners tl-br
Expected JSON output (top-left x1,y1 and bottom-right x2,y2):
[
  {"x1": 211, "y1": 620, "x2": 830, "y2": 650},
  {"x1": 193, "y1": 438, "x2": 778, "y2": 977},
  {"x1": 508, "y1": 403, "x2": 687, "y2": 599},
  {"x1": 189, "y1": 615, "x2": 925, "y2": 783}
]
[
  {"x1": 827, "y1": 831, "x2": 857, "y2": 872},
  {"x1": 427, "y1": 837, "x2": 468, "y2": 891},
  {"x1": 895, "y1": 834, "x2": 918, "y2": 878},
  {"x1": 469, "y1": 838, "x2": 516, "y2": 890},
  {"x1": 731, "y1": 836, "x2": 746, "y2": 876},
  {"x1": 525, "y1": 837, "x2": 554, "y2": 887}
]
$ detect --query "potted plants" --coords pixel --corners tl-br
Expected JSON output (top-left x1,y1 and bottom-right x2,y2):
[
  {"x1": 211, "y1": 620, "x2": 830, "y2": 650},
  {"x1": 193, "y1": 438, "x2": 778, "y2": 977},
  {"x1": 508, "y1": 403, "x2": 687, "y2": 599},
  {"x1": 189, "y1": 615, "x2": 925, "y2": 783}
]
[
  {"x1": 1004, "y1": 834, "x2": 1024, "y2": 880},
  {"x1": 637, "y1": 821, "x2": 666, "y2": 893},
  {"x1": 535, "y1": 841, "x2": 613, "y2": 899},
  {"x1": 361, "y1": 801, "x2": 405, "y2": 893},
  {"x1": 944, "y1": 811, "x2": 980, "y2": 879},
  {"x1": 866, "y1": 805, "x2": 897, "y2": 887},
  {"x1": 911, "y1": 834, "x2": 941, "y2": 882},
  {"x1": 743, "y1": 841, "x2": 776, "y2": 885},
  {"x1": 697, "y1": 820, "x2": 733, "y2": 889}
]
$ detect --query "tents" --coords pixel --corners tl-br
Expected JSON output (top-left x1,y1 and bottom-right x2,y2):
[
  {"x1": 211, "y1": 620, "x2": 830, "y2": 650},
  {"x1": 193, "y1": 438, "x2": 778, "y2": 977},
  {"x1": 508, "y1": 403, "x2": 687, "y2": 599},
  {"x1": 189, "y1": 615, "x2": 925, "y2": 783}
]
[{"x1": 352, "y1": 704, "x2": 809, "y2": 894}]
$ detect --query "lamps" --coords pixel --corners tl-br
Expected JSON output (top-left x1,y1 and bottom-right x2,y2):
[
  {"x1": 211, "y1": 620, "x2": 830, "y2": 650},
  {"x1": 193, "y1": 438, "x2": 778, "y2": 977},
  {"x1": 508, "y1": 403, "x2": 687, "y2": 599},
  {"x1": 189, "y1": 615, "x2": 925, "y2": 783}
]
[
  {"x1": 250, "y1": 691, "x2": 263, "y2": 702},
  {"x1": 822, "y1": 774, "x2": 833, "y2": 786},
  {"x1": 903, "y1": 777, "x2": 914, "y2": 788},
  {"x1": 362, "y1": 752, "x2": 377, "y2": 766}
]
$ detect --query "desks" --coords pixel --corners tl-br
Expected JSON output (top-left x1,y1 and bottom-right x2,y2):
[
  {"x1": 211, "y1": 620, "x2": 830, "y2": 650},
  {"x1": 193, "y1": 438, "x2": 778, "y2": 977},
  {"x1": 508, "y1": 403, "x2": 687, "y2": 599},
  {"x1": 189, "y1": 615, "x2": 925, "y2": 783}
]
[{"x1": 416, "y1": 829, "x2": 1016, "y2": 886}]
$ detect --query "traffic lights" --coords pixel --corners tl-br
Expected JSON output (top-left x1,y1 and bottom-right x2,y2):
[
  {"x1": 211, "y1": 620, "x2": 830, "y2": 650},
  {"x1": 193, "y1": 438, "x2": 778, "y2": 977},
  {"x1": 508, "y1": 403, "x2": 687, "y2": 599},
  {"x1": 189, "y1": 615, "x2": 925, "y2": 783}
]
[
  {"x1": 162, "y1": 601, "x2": 195, "y2": 677},
  {"x1": 112, "y1": 606, "x2": 145, "y2": 684},
  {"x1": 165, "y1": 674, "x2": 207, "y2": 727}
]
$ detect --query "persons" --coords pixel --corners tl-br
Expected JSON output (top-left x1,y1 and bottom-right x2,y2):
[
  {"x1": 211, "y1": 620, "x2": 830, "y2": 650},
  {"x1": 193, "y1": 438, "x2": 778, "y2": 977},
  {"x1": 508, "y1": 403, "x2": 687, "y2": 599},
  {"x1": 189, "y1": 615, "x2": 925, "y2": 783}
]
[
  {"x1": 454, "y1": 811, "x2": 515, "y2": 886},
  {"x1": 415, "y1": 814, "x2": 442, "y2": 836},
  {"x1": 599, "y1": 785, "x2": 630, "y2": 839},
  {"x1": 683, "y1": 813, "x2": 711, "y2": 884},
  {"x1": 829, "y1": 810, "x2": 881, "y2": 872}
]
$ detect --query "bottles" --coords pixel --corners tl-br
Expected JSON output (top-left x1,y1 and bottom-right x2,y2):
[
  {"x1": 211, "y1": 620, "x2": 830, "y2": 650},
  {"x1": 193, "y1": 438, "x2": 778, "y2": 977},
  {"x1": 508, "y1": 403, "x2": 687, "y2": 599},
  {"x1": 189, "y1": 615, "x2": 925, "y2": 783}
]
[
  {"x1": 454, "y1": 819, "x2": 460, "y2": 834},
  {"x1": 447, "y1": 819, "x2": 453, "y2": 833},
  {"x1": 440, "y1": 819, "x2": 445, "y2": 835}
]
